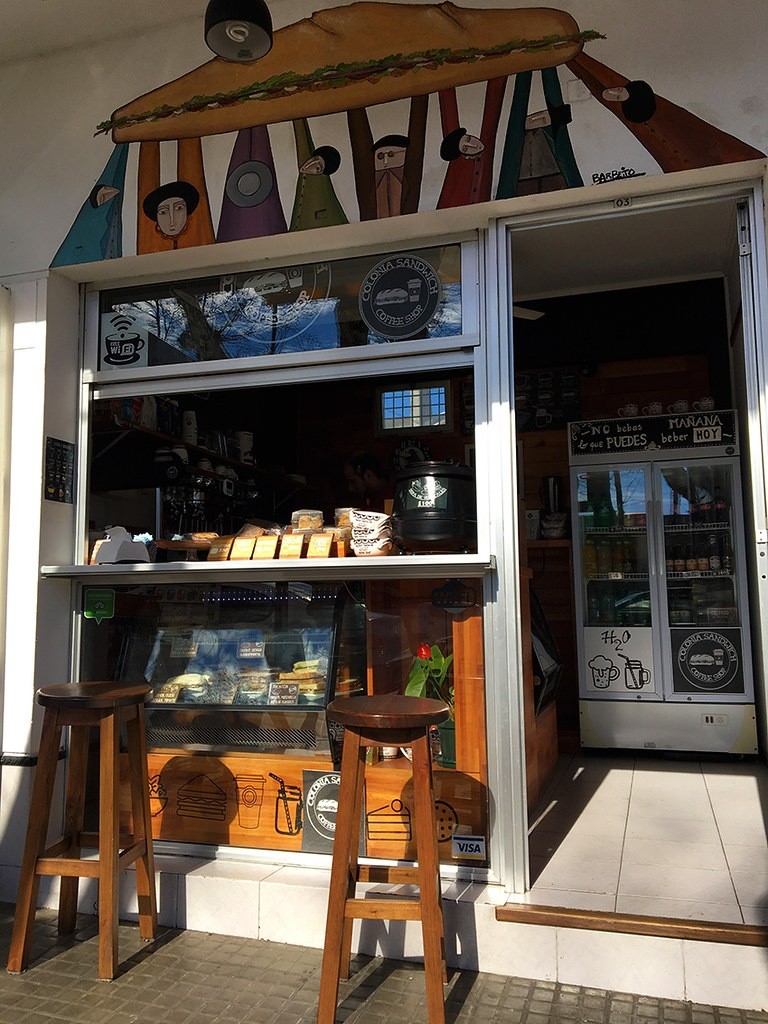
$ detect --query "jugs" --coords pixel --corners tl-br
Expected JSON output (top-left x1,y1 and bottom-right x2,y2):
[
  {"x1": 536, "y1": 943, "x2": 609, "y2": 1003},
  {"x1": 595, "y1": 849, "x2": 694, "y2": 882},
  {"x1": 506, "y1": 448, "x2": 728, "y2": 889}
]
[{"x1": 539, "y1": 475, "x2": 563, "y2": 514}]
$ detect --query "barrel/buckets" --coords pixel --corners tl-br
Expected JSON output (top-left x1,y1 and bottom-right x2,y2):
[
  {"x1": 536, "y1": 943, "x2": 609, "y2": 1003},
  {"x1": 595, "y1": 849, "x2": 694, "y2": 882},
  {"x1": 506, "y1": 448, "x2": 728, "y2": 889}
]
[{"x1": 390, "y1": 463, "x2": 477, "y2": 553}]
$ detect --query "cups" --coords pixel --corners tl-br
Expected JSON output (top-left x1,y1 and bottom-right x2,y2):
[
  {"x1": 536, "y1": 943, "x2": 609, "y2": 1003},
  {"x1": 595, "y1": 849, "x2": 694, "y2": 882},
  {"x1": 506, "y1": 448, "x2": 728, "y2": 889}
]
[
  {"x1": 693, "y1": 396, "x2": 715, "y2": 411},
  {"x1": 617, "y1": 403, "x2": 638, "y2": 417},
  {"x1": 642, "y1": 402, "x2": 662, "y2": 416},
  {"x1": 668, "y1": 400, "x2": 690, "y2": 414}
]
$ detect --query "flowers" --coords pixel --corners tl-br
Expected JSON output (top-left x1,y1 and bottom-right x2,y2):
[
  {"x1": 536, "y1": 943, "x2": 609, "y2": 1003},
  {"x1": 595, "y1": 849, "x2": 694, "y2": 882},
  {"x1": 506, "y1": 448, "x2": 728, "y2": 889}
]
[{"x1": 404, "y1": 638, "x2": 455, "y2": 723}]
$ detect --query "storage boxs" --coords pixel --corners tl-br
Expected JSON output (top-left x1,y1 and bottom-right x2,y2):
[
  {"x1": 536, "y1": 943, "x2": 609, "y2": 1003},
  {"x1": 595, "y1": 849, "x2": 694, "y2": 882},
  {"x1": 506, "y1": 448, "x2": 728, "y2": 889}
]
[
  {"x1": 93, "y1": 395, "x2": 180, "y2": 437},
  {"x1": 525, "y1": 509, "x2": 547, "y2": 540}
]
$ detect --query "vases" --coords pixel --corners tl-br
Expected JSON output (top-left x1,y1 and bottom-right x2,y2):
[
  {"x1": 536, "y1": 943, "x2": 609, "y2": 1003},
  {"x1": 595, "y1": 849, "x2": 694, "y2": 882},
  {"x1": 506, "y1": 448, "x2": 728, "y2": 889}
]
[
  {"x1": 434, "y1": 722, "x2": 456, "y2": 768},
  {"x1": 378, "y1": 747, "x2": 398, "y2": 761}
]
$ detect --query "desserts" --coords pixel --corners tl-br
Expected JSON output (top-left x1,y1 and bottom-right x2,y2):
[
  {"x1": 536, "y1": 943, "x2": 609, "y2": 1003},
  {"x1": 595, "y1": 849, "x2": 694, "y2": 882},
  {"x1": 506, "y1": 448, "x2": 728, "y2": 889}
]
[{"x1": 191, "y1": 509, "x2": 351, "y2": 540}]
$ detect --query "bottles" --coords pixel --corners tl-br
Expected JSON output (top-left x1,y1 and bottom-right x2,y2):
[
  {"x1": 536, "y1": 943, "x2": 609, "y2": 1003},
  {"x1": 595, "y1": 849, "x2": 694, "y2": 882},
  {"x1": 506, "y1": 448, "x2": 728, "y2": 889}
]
[{"x1": 579, "y1": 492, "x2": 738, "y2": 626}]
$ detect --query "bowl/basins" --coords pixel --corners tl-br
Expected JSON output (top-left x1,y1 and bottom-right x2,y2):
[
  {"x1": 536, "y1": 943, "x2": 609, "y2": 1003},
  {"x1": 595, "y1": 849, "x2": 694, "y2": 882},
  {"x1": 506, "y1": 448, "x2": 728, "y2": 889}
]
[{"x1": 349, "y1": 509, "x2": 395, "y2": 557}]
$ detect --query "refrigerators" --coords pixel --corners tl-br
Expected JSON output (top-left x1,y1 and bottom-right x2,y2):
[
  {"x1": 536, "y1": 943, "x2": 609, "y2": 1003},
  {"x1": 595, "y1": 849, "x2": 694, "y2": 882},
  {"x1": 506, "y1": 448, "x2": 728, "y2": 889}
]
[{"x1": 565, "y1": 408, "x2": 759, "y2": 760}]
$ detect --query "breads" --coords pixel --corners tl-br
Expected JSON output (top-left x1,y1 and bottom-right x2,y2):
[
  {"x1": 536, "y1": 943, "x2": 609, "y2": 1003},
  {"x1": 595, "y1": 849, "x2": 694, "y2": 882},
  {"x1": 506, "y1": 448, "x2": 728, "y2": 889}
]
[{"x1": 167, "y1": 660, "x2": 326, "y2": 700}]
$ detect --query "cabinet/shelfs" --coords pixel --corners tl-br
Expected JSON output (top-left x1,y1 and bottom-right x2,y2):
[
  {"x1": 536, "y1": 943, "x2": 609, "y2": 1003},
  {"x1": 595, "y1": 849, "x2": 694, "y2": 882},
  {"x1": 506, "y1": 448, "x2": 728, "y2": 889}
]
[
  {"x1": 81, "y1": 587, "x2": 490, "y2": 868},
  {"x1": 527, "y1": 567, "x2": 565, "y2": 798},
  {"x1": 92, "y1": 415, "x2": 309, "y2": 519}
]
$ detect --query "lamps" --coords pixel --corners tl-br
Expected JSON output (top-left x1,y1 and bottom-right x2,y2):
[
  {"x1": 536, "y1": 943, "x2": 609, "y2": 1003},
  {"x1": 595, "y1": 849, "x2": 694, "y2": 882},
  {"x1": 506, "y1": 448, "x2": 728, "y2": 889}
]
[{"x1": 204, "y1": 0, "x2": 273, "y2": 63}]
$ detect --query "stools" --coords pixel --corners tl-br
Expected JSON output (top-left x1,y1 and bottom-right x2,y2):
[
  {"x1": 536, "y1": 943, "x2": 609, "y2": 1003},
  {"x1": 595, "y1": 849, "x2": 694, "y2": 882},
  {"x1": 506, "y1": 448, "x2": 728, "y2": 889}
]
[
  {"x1": 5, "y1": 681, "x2": 159, "y2": 982},
  {"x1": 316, "y1": 695, "x2": 450, "y2": 1024}
]
